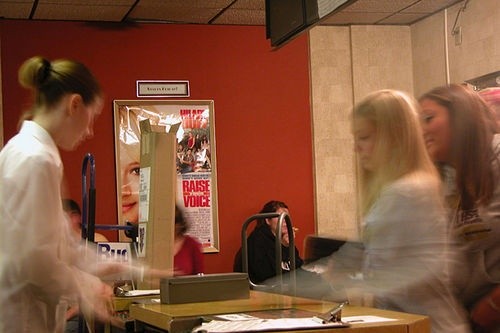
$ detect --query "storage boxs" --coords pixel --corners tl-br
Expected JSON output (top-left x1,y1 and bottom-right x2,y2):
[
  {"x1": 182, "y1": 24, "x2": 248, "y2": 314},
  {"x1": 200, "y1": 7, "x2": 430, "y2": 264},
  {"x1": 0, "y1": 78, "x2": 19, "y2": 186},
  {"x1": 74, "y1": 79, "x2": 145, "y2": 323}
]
[
  {"x1": 135, "y1": 119, "x2": 183, "y2": 289},
  {"x1": 159, "y1": 272, "x2": 250, "y2": 305}
]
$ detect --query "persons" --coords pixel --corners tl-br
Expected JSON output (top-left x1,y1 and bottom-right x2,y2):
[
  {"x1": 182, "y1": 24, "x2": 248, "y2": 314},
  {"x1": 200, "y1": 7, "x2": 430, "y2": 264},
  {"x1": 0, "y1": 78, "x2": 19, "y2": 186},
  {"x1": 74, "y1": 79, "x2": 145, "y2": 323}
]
[
  {"x1": 246, "y1": 89, "x2": 474, "y2": 333},
  {"x1": 417, "y1": 82, "x2": 500, "y2": 333},
  {"x1": 1, "y1": 57, "x2": 183, "y2": 333},
  {"x1": 59, "y1": 198, "x2": 111, "y2": 333},
  {"x1": 171, "y1": 204, "x2": 205, "y2": 278},
  {"x1": 119, "y1": 102, "x2": 215, "y2": 246},
  {"x1": 233, "y1": 199, "x2": 304, "y2": 291}
]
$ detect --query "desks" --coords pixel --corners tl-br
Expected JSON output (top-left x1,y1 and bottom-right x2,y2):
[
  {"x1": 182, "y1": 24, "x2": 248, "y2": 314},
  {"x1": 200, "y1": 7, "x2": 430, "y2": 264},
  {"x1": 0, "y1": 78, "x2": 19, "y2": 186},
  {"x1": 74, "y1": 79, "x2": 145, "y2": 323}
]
[{"x1": 77, "y1": 287, "x2": 431, "y2": 333}]
